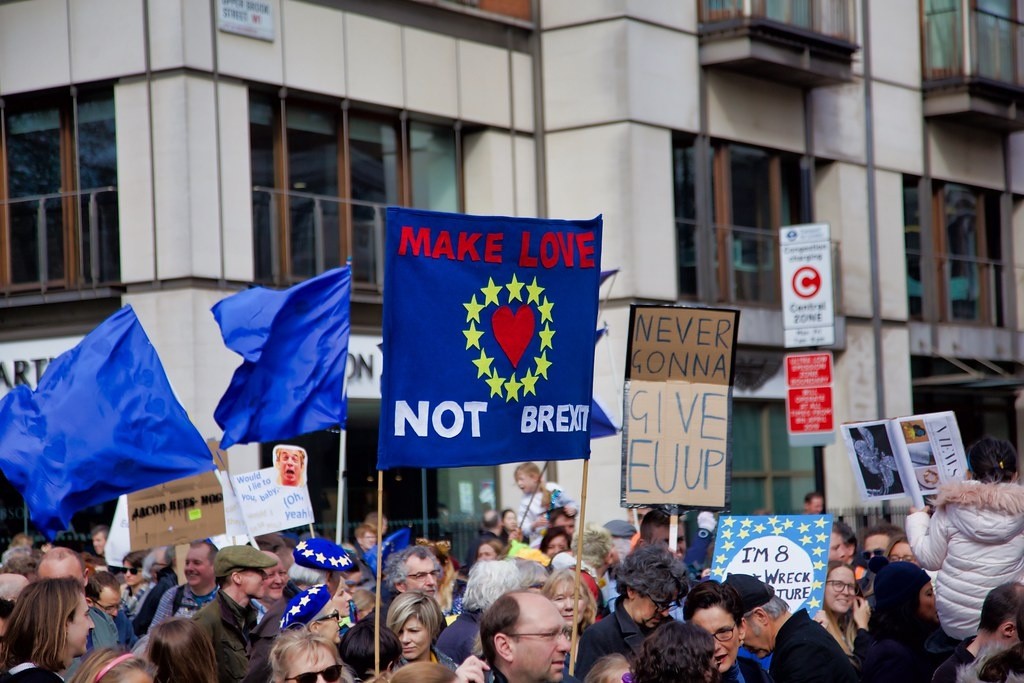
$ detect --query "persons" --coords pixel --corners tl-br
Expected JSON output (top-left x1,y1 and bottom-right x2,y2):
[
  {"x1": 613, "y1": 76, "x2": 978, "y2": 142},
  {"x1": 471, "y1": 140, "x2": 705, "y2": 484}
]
[
  {"x1": 271, "y1": 445, "x2": 306, "y2": 485},
  {"x1": 0, "y1": 437, "x2": 1024, "y2": 682}
]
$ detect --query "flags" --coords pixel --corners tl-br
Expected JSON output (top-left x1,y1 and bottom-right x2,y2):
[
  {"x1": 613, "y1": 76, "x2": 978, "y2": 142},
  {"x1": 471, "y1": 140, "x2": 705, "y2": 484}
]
[
  {"x1": 210, "y1": 255, "x2": 348, "y2": 451},
  {"x1": 0, "y1": 304, "x2": 218, "y2": 543}
]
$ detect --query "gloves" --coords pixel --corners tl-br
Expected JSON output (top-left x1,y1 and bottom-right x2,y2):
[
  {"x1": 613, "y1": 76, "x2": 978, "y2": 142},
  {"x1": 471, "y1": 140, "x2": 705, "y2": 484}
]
[{"x1": 698, "y1": 511, "x2": 718, "y2": 532}]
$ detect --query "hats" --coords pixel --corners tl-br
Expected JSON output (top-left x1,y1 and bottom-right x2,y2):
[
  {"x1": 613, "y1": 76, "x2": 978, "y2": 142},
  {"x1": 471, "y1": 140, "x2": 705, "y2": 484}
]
[
  {"x1": 214, "y1": 545, "x2": 278, "y2": 578},
  {"x1": 720, "y1": 573, "x2": 775, "y2": 613},
  {"x1": 603, "y1": 519, "x2": 636, "y2": 538},
  {"x1": 292, "y1": 538, "x2": 353, "y2": 571},
  {"x1": 873, "y1": 561, "x2": 932, "y2": 610},
  {"x1": 279, "y1": 583, "x2": 331, "y2": 632}
]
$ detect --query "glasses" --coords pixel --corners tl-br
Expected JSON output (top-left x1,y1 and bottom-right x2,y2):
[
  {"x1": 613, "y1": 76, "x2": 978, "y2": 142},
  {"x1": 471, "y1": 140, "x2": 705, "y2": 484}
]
[
  {"x1": 345, "y1": 578, "x2": 369, "y2": 588},
  {"x1": 824, "y1": 579, "x2": 859, "y2": 596},
  {"x1": 316, "y1": 610, "x2": 340, "y2": 621},
  {"x1": 646, "y1": 592, "x2": 681, "y2": 611},
  {"x1": 284, "y1": 664, "x2": 343, "y2": 683},
  {"x1": 122, "y1": 566, "x2": 143, "y2": 576},
  {"x1": 89, "y1": 597, "x2": 126, "y2": 614},
  {"x1": 0, "y1": 598, "x2": 16, "y2": 618},
  {"x1": 861, "y1": 548, "x2": 885, "y2": 561},
  {"x1": 504, "y1": 621, "x2": 573, "y2": 642},
  {"x1": 408, "y1": 569, "x2": 443, "y2": 582},
  {"x1": 711, "y1": 623, "x2": 738, "y2": 642}
]
